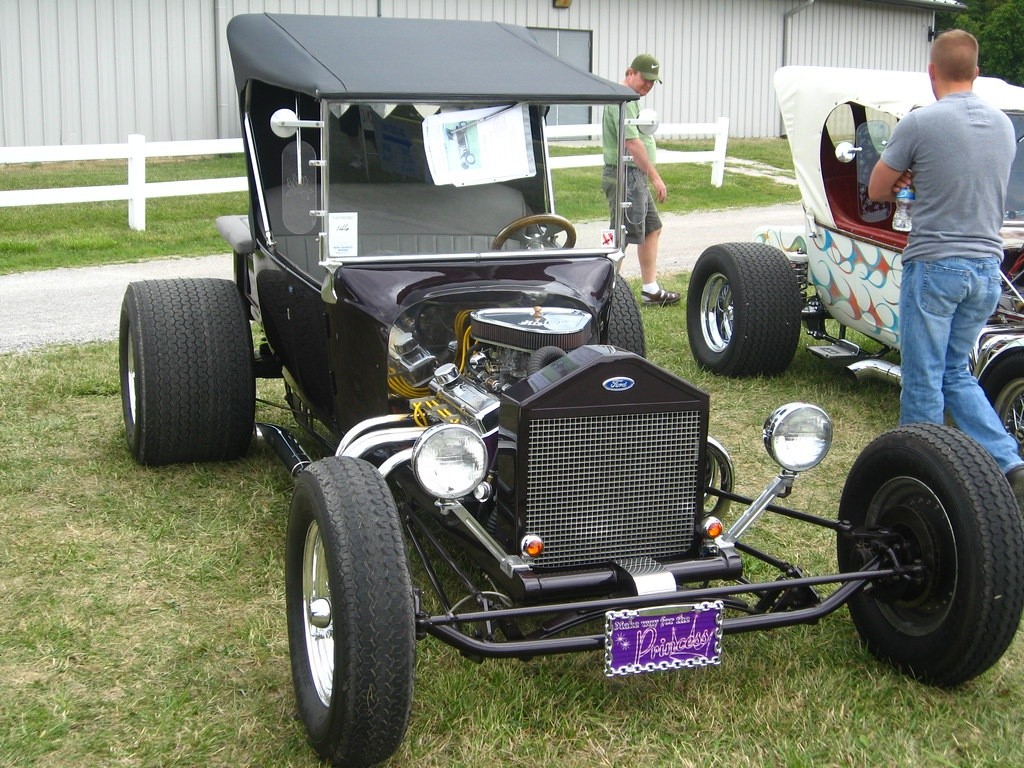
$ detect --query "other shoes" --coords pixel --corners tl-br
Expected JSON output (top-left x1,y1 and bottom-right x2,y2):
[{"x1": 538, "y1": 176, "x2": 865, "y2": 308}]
[{"x1": 1005, "y1": 463, "x2": 1024, "y2": 504}]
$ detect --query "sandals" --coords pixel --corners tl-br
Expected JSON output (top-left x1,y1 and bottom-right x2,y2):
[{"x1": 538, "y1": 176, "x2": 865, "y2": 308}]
[{"x1": 640, "y1": 288, "x2": 682, "y2": 304}]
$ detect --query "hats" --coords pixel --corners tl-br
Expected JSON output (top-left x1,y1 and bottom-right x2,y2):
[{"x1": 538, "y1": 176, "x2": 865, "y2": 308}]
[{"x1": 630, "y1": 52, "x2": 663, "y2": 85}]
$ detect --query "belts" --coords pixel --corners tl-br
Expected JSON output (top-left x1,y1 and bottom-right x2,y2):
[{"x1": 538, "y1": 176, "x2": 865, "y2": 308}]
[{"x1": 605, "y1": 163, "x2": 640, "y2": 171}]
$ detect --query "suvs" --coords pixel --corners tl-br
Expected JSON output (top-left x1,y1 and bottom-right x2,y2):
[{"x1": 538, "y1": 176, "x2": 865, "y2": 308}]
[{"x1": 121, "y1": 12, "x2": 1024, "y2": 768}]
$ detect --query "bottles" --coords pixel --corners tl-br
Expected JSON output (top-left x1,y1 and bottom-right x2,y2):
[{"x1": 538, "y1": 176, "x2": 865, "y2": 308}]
[{"x1": 892, "y1": 168, "x2": 915, "y2": 232}]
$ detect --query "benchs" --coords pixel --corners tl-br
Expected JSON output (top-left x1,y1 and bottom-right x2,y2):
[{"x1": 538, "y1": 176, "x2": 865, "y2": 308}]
[{"x1": 257, "y1": 183, "x2": 538, "y2": 285}]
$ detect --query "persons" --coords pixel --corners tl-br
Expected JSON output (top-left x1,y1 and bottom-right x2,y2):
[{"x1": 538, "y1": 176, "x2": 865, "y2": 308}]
[
  {"x1": 870, "y1": 29, "x2": 1023, "y2": 514},
  {"x1": 601, "y1": 54, "x2": 682, "y2": 309}
]
